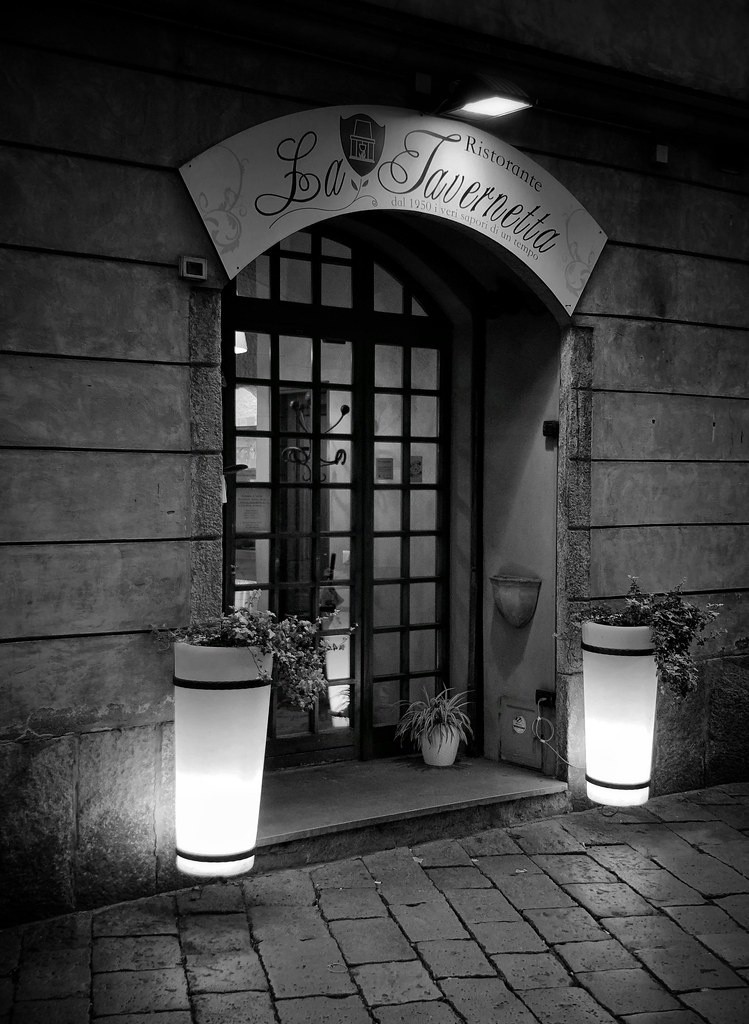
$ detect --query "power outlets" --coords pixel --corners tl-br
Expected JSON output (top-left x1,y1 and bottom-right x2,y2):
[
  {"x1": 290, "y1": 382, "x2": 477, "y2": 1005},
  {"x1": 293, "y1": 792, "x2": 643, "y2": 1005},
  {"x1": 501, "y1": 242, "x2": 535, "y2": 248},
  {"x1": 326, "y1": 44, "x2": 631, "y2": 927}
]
[{"x1": 535, "y1": 688, "x2": 557, "y2": 712}]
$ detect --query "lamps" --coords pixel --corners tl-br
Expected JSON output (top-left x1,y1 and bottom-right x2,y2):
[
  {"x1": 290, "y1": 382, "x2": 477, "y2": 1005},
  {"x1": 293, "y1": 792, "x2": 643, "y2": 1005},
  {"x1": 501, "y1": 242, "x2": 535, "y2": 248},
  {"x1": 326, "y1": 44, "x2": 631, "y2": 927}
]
[
  {"x1": 580, "y1": 650, "x2": 659, "y2": 807},
  {"x1": 174, "y1": 685, "x2": 270, "y2": 876}
]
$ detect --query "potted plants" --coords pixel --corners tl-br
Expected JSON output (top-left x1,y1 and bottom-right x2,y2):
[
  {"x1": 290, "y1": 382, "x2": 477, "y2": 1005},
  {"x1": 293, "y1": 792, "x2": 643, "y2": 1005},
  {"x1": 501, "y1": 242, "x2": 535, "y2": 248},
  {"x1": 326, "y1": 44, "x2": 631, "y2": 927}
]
[
  {"x1": 553, "y1": 574, "x2": 728, "y2": 700},
  {"x1": 149, "y1": 589, "x2": 360, "y2": 712},
  {"x1": 389, "y1": 682, "x2": 475, "y2": 767}
]
